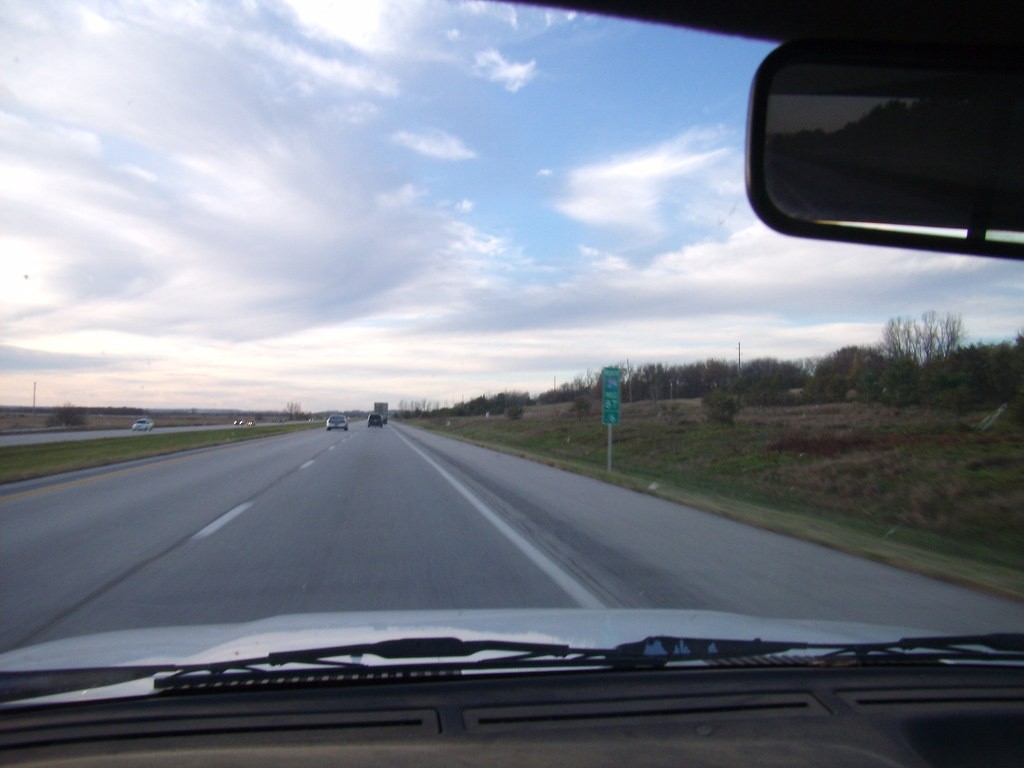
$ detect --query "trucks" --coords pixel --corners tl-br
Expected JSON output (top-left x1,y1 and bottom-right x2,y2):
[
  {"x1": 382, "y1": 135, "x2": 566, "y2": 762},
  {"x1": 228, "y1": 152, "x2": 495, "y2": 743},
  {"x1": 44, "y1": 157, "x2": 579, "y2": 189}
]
[{"x1": 374, "y1": 402, "x2": 388, "y2": 424}]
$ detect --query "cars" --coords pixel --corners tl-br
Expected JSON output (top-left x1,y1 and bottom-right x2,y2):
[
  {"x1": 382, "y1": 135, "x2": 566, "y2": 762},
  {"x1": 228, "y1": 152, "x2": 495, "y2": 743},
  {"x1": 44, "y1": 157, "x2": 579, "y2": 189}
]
[
  {"x1": 326, "y1": 414, "x2": 350, "y2": 431},
  {"x1": 232, "y1": 419, "x2": 256, "y2": 427},
  {"x1": 132, "y1": 418, "x2": 154, "y2": 431}
]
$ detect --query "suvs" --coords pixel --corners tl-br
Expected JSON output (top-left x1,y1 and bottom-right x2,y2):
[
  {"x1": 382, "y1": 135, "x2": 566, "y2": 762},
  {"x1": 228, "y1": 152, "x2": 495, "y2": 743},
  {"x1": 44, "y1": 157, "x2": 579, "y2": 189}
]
[{"x1": 366, "y1": 414, "x2": 384, "y2": 427}]
